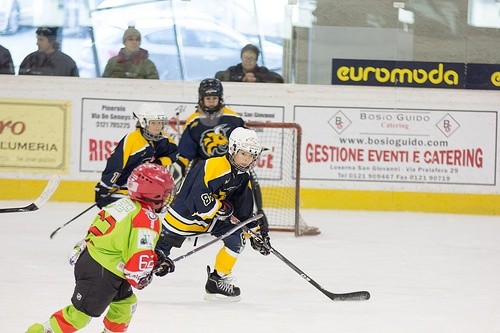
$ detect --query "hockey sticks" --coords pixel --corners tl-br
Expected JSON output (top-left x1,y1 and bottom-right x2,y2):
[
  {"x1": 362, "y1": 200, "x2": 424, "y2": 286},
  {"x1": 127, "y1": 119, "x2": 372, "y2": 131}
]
[
  {"x1": 229, "y1": 210, "x2": 372, "y2": 302},
  {"x1": 48, "y1": 189, "x2": 124, "y2": 244},
  {"x1": 145, "y1": 213, "x2": 265, "y2": 276},
  {"x1": 0, "y1": 175, "x2": 60, "y2": 214}
]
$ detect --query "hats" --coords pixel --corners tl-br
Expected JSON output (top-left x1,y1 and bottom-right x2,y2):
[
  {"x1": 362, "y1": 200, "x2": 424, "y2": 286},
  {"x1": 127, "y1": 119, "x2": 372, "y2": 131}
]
[
  {"x1": 36, "y1": 27, "x2": 58, "y2": 37},
  {"x1": 123, "y1": 28, "x2": 141, "y2": 45}
]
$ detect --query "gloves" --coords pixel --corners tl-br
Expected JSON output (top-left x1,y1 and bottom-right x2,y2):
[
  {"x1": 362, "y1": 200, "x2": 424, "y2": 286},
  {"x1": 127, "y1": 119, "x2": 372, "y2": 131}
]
[
  {"x1": 95, "y1": 184, "x2": 110, "y2": 210},
  {"x1": 155, "y1": 250, "x2": 175, "y2": 276},
  {"x1": 250, "y1": 232, "x2": 271, "y2": 255},
  {"x1": 215, "y1": 200, "x2": 234, "y2": 218}
]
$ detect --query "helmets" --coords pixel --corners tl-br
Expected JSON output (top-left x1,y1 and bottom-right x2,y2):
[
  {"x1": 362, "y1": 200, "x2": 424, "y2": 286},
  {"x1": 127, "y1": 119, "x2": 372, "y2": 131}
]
[
  {"x1": 198, "y1": 78, "x2": 222, "y2": 113},
  {"x1": 134, "y1": 106, "x2": 168, "y2": 142},
  {"x1": 128, "y1": 164, "x2": 175, "y2": 213},
  {"x1": 228, "y1": 127, "x2": 262, "y2": 171}
]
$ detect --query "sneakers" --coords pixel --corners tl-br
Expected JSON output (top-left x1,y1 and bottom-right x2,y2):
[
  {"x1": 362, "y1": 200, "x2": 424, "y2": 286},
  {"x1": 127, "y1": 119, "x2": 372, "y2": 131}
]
[{"x1": 205, "y1": 265, "x2": 241, "y2": 302}]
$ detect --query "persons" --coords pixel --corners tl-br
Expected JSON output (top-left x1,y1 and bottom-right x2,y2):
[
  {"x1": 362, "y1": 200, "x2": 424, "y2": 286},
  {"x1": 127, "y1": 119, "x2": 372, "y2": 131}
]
[
  {"x1": 101, "y1": 25, "x2": 159, "y2": 79},
  {"x1": 18, "y1": 25, "x2": 79, "y2": 77},
  {"x1": 25, "y1": 164, "x2": 177, "y2": 333},
  {"x1": 94, "y1": 108, "x2": 189, "y2": 213},
  {"x1": 214, "y1": 44, "x2": 284, "y2": 83},
  {"x1": 0, "y1": 45, "x2": 15, "y2": 75},
  {"x1": 156, "y1": 127, "x2": 271, "y2": 302},
  {"x1": 178, "y1": 77, "x2": 268, "y2": 233}
]
